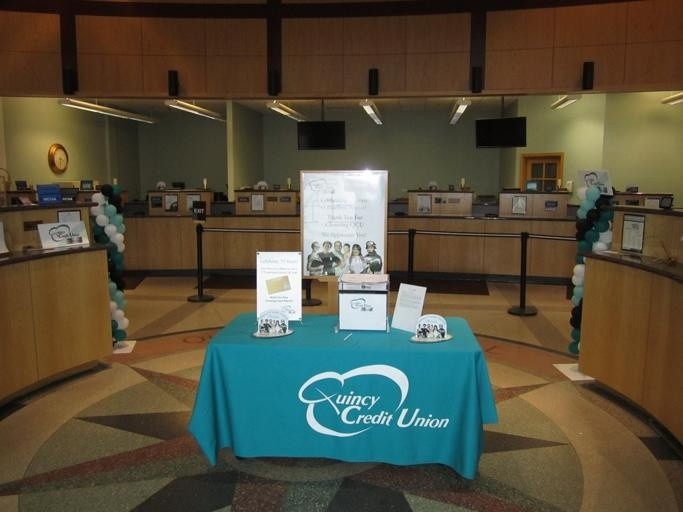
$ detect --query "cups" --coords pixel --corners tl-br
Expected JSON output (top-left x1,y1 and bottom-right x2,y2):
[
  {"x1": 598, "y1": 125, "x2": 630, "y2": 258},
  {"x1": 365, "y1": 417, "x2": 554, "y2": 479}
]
[
  {"x1": 201, "y1": 177, "x2": 208, "y2": 190},
  {"x1": 273, "y1": 184, "x2": 281, "y2": 189},
  {"x1": 448, "y1": 185, "x2": 454, "y2": 191}
]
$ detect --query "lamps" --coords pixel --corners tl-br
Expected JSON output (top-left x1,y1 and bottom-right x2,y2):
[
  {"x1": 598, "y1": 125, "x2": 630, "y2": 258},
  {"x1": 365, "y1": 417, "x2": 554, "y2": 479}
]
[
  {"x1": 447, "y1": 98, "x2": 471, "y2": 126},
  {"x1": 269, "y1": 102, "x2": 314, "y2": 123},
  {"x1": 63, "y1": 98, "x2": 155, "y2": 124},
  {"x1": 551, "y1": 94, "x2": 581, "y2": 109},
  {"x1": 164, "y1": 100, "x2": 226, "y2": 122},
  {"x1": 661, "y1": 91, "x2": 683, "y2": 105},
  {"x1": 360, "y1": 102, "x2": 383, "y2": 125}
]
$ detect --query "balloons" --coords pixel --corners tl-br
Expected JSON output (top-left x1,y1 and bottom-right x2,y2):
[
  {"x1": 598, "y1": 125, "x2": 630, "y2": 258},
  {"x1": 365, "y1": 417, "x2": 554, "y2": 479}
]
[
  {"x1": 569, "y1": 185, "x2": 614, "y2": 353},
  {"x1": 90, "y1": 183, "x2": 129, "y2": 348}
]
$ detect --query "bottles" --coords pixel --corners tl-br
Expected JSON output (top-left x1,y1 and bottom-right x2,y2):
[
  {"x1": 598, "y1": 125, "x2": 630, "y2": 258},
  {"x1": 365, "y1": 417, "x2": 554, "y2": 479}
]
[{"x1": 286, "y1": 177, "x2": 292, "y2": 190}]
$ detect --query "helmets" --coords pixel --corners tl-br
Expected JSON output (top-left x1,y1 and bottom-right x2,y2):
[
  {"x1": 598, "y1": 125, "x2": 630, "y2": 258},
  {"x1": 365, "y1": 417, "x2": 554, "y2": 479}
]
[{"x1": 365, "y1": 240, "x2": 376, "y2": 249}]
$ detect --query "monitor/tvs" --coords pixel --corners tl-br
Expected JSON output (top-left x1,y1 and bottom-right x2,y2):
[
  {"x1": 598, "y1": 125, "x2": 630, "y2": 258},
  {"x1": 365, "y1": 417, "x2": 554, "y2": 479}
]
[
  {"x1": 297, "y1": 121, "x2": 345, "y2": 150},
  {"x1": 476, "y1": 116, "x2": 526, "y2": 148}
]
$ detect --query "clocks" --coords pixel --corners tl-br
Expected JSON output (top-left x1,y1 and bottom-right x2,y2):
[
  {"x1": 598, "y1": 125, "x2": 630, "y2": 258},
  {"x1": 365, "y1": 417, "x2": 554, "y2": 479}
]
[{"x1": 48, "y1": 143, "x2": 69, "y2": 173}]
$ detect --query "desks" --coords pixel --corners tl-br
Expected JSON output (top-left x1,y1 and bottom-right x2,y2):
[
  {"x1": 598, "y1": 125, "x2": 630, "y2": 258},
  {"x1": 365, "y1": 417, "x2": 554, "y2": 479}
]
[{"x1": 189, "y1": 312, "x2": 499, "y2": 480}]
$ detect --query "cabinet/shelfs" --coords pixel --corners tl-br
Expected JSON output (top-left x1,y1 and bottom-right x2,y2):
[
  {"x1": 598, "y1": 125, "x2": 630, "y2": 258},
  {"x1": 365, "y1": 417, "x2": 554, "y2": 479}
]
[
  {"x1": 1, "y1": 10, "x2": 66, "y2": 99},
  {"x1": 480, "y1": 6, "x2": 683, "y2": 96},
  {"x1": 273, "y1": 11, "x2": 478, "y2": 97},
  {"x1": 69, "y1": 12, "x2": 270, "y2": 103}
]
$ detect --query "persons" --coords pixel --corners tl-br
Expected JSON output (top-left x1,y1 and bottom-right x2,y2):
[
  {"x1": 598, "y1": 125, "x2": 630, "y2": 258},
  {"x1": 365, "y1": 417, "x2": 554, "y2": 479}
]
[
  {"x1": 259, "y1": 318, "x2": 288, "y2": 334},
  {"x1": 416, "y1": 323, "x2": 446, "y2": 339},
  {"x1": 317, "y1": 241, "x2": 341, "y2": 276},
  {"x1": 306, "y1": 242, "x2": 324, "y2": 276},
  {"x1": 342, "y1": 243, "x2": 350, "y2": 273},
  {"x1": 348, "y1": 244, "x2": 366, "y2": 274},
  {"x1": 363, "y1": 241, "x2": 382, "y2": 275},
  {"x1": 332, "y1": 241, "x2": 344, "y2": 275}
]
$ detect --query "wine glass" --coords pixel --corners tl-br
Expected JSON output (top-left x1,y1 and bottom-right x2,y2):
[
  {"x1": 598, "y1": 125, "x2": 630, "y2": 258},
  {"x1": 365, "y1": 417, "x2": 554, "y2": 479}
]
[{"x1": 458, "y1": 174, "x2": 473, "y2": 192}]
